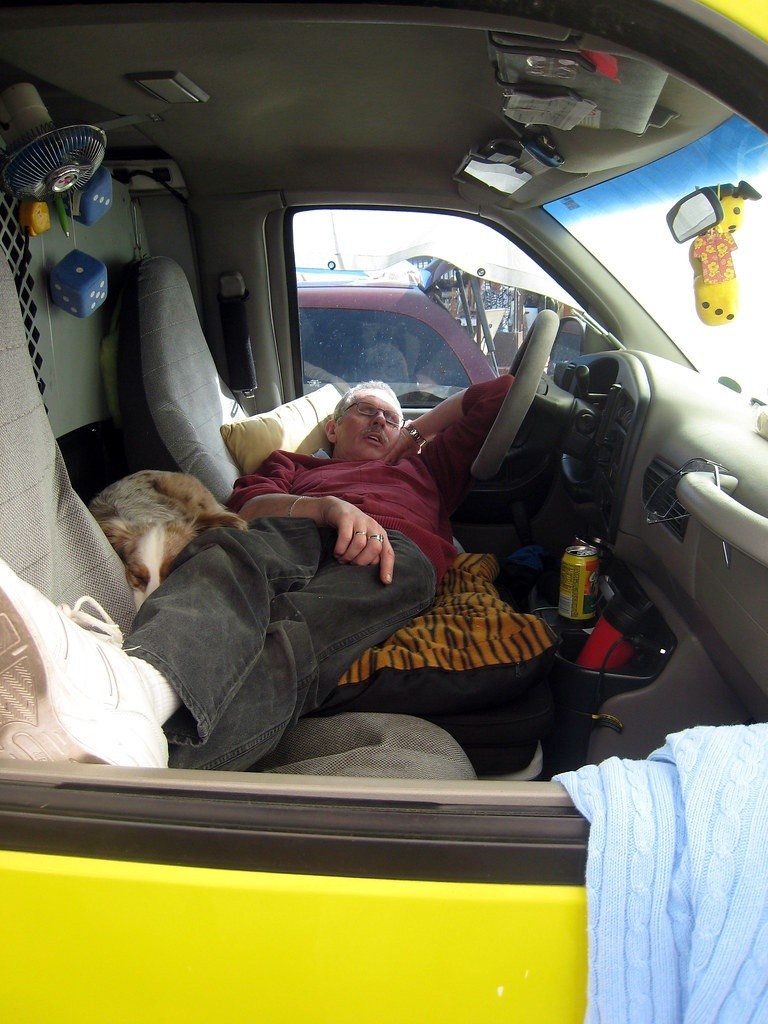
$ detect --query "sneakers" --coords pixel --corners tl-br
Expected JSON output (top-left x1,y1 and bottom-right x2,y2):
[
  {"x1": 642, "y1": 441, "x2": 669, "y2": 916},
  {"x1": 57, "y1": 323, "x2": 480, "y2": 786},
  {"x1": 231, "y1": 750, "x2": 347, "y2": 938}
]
[{"x1": 0, "y1": 557, "x2": 169, "y2": 770}]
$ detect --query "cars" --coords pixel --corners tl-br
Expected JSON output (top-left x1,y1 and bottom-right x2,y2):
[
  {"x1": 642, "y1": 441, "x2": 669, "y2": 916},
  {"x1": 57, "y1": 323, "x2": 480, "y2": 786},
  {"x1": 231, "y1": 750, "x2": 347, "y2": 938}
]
[{"x1": 296, "y1": 279, "x2": 499, "y2": 413}]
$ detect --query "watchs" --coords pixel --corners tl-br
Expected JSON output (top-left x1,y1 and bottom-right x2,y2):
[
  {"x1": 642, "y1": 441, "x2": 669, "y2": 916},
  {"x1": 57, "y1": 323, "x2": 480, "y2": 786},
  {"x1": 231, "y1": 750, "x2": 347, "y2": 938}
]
[{"x1": 403, "y1": 419, "x2": 426, "y2": 447}]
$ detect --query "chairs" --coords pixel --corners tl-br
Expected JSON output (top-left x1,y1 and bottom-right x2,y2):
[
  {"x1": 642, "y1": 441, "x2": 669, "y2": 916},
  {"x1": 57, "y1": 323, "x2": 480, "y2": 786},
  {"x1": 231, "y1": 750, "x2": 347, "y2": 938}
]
[
  {"x1": 0, "y1": 245, "x2": 482, "y2": 783},
  {"x1": 135, "y1": 258, "x2": 243, "y2": 496}
]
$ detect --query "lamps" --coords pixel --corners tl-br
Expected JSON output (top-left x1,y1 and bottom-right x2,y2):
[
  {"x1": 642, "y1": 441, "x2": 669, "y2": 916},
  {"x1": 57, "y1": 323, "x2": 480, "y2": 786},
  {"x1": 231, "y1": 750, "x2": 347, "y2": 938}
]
[{"x1": 128, "y1": 70, "x2": 210, "y2": 106}]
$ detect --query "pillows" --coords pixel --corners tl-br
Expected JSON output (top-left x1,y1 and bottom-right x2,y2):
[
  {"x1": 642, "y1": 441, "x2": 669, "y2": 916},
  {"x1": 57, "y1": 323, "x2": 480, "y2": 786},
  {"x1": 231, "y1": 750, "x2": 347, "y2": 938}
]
[{"x1": 217, "y1": 373, "x2": 343, "y2": 476}]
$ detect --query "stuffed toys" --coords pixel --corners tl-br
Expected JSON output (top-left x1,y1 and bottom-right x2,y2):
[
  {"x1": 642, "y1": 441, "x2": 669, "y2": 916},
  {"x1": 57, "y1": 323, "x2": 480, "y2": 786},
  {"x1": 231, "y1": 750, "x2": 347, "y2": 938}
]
[{"x1": 689, "y1": 180, "x2": 762, "y2": 326}]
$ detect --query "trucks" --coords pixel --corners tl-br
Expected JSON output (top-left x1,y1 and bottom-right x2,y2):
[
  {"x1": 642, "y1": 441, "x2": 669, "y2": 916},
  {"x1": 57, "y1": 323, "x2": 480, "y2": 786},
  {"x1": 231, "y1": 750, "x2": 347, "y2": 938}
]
[{"x1": 0, "y1": 0, "x2": 768, "y2": 1024}]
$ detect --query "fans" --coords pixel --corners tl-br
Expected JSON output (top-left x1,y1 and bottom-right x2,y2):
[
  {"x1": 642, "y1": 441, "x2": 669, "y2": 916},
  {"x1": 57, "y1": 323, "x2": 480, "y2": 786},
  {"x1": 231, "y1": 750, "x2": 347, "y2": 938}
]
[{"x1": 1, "y1": 83, "x2": 106, "y2": 200}]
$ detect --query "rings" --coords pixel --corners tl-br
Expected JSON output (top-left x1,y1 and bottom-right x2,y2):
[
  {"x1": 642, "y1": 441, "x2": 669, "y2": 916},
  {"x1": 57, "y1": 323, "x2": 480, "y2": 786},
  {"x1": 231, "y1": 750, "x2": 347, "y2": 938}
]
[
  {"x1": 367, "y1": 534, "x2": 384, "y2": 543},
  {"x1": 354, "y1": 532, "x2": 367, "y2": 535}
]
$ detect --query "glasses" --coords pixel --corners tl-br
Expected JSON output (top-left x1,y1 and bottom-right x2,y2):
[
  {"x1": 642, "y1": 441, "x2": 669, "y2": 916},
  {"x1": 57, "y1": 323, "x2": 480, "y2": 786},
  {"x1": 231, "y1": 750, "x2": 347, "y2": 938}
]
[{"x1": 335, "y1": 402, "x2": 406, "y2": 430}]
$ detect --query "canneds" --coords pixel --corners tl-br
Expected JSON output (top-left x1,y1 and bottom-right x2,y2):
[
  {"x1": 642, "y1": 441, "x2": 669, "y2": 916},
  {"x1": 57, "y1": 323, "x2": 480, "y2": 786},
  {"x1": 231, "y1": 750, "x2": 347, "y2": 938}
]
[{"x1": 557, "y1": 545, "x2": 600, "y2": 621}]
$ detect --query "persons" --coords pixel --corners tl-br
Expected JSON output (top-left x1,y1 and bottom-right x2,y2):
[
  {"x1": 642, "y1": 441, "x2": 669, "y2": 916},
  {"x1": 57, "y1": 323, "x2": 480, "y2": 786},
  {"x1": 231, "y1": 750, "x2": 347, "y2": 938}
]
[
  {"x1": 0, "y1": 374, "x2": 514, "y2": 772},
  {"x1": 483, "y1": 281, "x2": 520, "y2": 333}
]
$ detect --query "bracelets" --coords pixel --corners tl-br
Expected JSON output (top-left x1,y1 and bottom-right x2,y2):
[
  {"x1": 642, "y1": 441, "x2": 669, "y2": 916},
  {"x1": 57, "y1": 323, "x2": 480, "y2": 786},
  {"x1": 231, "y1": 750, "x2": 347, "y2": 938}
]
[{"x1": 288, "y1": 496, "x2": 306, "y2": 518}]
